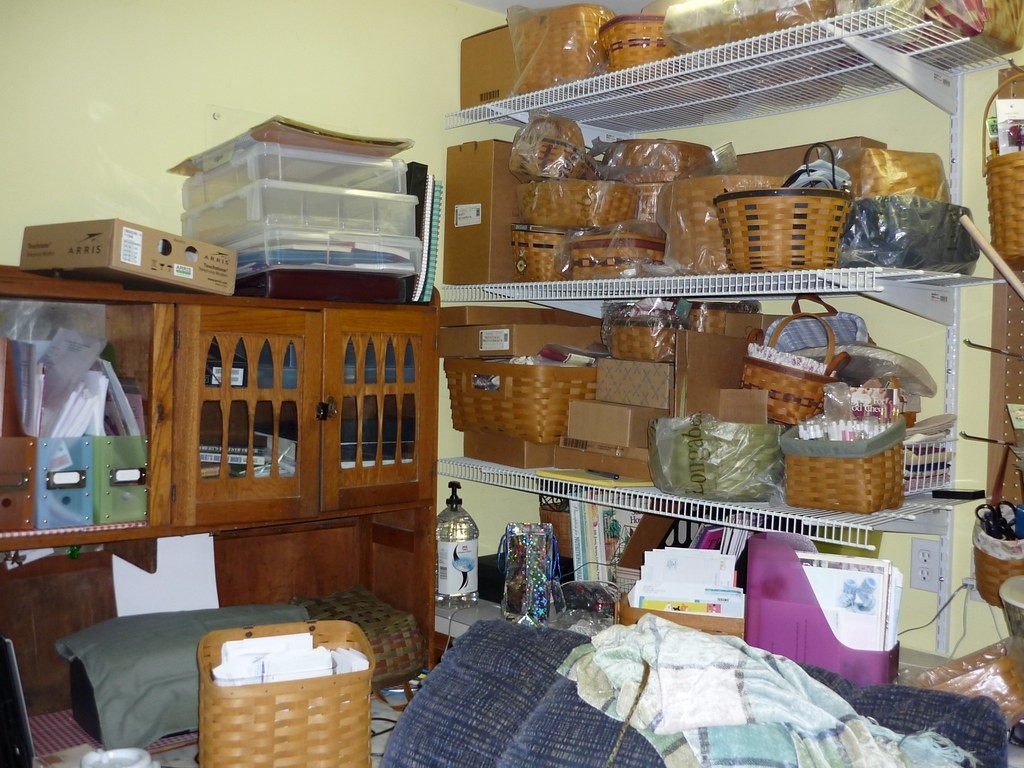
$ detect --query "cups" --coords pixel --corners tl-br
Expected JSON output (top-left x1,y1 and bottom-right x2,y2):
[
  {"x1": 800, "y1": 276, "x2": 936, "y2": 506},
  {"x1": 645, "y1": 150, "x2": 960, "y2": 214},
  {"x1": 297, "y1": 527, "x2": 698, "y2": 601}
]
[{"x1": 999, "y1": 574, "x2": 1024, "y2": 639}]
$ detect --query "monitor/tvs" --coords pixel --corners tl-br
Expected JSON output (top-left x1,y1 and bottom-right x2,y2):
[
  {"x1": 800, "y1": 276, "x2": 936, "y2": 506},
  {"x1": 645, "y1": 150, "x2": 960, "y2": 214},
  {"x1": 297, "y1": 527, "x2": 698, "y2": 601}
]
[{"x1": 0, "y1": 635, "x2": 36, "y2": 768}]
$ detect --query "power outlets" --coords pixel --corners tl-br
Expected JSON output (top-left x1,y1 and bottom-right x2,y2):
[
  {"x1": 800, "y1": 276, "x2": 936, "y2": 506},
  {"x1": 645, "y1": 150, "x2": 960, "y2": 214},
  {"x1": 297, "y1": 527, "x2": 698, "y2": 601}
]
[{"x1": 909, "y1": 535, "x2": 941, "y2": 593}]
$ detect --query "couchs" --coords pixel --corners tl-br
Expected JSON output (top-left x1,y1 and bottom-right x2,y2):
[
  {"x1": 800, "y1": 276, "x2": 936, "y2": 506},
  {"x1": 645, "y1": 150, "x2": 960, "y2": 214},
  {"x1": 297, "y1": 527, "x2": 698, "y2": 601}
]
[{"x1": 380, "y1": 618, "x2": 1008, "y2": 768}]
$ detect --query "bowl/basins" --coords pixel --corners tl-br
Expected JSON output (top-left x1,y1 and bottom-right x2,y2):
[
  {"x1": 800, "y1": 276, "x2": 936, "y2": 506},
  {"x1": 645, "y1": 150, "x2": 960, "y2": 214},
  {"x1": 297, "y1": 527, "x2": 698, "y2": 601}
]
[{"x1": 688, "y1": 142, "x2": 743, "y2": 178}]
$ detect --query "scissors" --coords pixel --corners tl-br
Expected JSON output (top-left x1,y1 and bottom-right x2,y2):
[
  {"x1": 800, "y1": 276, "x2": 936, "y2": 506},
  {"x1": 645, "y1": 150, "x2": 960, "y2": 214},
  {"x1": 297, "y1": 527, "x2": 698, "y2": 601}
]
[{"x1": 975, "y1": 502, "x2": 1016, "y2": 528}]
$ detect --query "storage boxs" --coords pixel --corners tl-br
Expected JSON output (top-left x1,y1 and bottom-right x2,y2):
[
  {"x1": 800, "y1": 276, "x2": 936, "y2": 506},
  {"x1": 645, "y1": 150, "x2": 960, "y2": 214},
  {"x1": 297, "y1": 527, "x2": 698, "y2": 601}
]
[
  {"x1": 19, "y1": 218, "x2": 238, "y2": 298},
  {"x1": 180, "y1": 141, "x2": 408, "y2": 212},
  {"x1": 459, "y1": 24, "x2": 527, "y2": 110},
  {"x1": 441, "y1": 138, "x2": 527, "y2": 285},
  {"x1": 215, "y1": 222, "x2": 424, "y2": 279},
  {"x1": 737, "y1": 135, "x2": 887, "y2": 197},
  {"x1": 438, "y1": 306, "x2": 771, "y2": 480},
  {"x1": 619, "y1": 593, "x2": 745, "y2": 639},
  {"x1": 180, "y1": 179, "x2": 419, "y2": 246}
]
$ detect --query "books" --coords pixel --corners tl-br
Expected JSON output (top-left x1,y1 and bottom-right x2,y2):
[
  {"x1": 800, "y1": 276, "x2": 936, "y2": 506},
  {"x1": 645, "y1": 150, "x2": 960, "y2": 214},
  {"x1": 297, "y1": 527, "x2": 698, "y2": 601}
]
[
  {"x1": 627, "y1": 543, "x2": 744, "y2": 619},
  {"x1": 9, "y1": 325, "x2": 148, "y2": 437},
  {"x1": 404, "y1": 159, "x2": 444, "y2": 305},
  {"x1": 689, "y1": 513, "x2": 765, "y2": 593},
  {"x1": 192, "y1": 431, "x2": 269, "y2": 477},
  {"x1": 568, "y1": 490, "x2": 642, "y2": 587},
  {"x1": 616, "y1": 499, "x2": 708, "y2": 585},
  {"x1": 795, "y1": 547, "x2": 902, "y2": 653}
]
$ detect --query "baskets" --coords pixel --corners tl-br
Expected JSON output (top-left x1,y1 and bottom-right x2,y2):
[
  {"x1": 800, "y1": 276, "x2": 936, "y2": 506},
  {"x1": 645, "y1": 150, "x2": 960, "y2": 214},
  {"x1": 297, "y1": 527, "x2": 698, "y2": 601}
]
[
  {"x1": 440, "y1": 1, "x2": 1024, "y2": 513},
  {"x1": 972, "y1": 521, "x2": 1024, "y2": 608},
  {"x1": 293, "y1": 583, "x2": 425, "y2": 692},
  {"x1": 198, "y1": 619, "x2": 375, "y2": 768}
]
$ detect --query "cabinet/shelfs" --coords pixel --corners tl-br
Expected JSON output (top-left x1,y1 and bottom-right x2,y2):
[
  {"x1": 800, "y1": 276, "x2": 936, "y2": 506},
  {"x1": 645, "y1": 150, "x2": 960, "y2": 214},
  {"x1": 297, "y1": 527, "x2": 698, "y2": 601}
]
[
  {"x1": 435, "y1": 0, "x2": 1012, "y2": 679},
  {"x1": 0, "y1": 279, "x2": 442, "y2": 757}
]
